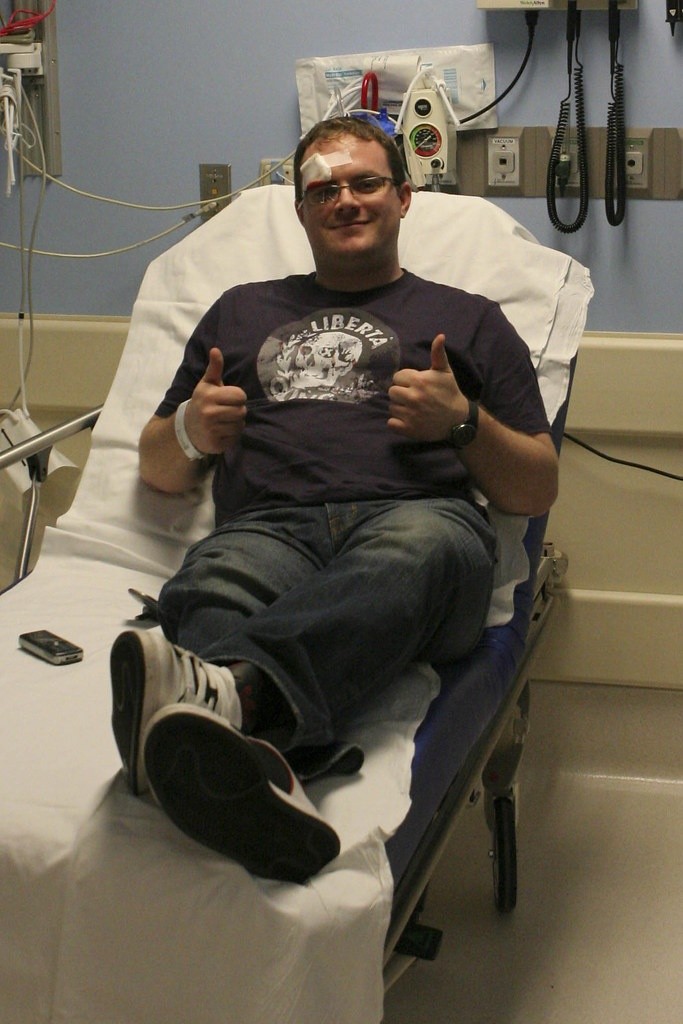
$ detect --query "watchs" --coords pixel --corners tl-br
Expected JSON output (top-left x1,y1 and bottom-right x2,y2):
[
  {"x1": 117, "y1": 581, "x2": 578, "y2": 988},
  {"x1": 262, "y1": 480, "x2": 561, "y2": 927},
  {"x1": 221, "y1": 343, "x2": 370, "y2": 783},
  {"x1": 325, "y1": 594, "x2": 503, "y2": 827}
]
[{"x1": 447, "y1": 401, "x2": 480, "y2": 449}]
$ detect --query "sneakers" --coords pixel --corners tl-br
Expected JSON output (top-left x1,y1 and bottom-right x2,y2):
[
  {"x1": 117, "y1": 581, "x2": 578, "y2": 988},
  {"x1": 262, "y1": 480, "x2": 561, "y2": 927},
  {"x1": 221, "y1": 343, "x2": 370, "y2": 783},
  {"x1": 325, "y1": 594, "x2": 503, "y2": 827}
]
[
  {"x1": 109, "y1": 629, "x2": 244, "y2": 797},
  {"x1": 141, "y1": 702, "x2": 341, "y2": 886}
]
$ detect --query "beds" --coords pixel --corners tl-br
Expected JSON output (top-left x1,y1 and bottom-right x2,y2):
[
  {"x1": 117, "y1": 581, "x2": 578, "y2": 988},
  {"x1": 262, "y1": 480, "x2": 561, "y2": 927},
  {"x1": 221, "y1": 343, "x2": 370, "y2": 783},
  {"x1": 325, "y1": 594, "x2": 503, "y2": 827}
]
[{"x1": 0, "y1": 185, "x2": 596, "y2": 1024}]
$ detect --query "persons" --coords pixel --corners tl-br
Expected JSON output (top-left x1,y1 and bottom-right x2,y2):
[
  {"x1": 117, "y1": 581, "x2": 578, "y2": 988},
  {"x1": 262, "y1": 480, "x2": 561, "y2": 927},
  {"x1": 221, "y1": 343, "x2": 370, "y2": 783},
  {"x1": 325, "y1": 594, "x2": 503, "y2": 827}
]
[{"x1": 105, "y1": 114, "x2": 559, "y2": 881}]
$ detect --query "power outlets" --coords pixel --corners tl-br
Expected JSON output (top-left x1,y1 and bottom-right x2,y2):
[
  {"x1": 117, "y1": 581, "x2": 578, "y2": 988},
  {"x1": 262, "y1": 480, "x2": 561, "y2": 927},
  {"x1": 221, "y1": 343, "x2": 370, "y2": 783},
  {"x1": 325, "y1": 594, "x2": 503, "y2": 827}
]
[{"x1": 258, "y1": 158, "x2": 295, "y2": 186}]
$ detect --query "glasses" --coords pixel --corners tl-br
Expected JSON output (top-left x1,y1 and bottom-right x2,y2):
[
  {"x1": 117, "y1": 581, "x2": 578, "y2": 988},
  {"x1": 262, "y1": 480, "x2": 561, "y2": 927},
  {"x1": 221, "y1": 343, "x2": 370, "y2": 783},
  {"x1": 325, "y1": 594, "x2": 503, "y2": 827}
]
[{"x1": 297, "y1": 175, "x2": 396, "y2": 211}]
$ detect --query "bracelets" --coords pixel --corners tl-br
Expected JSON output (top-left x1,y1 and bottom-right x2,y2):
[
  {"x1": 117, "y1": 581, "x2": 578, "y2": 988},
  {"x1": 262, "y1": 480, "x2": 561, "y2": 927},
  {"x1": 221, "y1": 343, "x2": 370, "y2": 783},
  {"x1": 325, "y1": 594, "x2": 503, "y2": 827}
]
[{"x1": 175, "y1": 400, "x2": 206, "y2": 461}]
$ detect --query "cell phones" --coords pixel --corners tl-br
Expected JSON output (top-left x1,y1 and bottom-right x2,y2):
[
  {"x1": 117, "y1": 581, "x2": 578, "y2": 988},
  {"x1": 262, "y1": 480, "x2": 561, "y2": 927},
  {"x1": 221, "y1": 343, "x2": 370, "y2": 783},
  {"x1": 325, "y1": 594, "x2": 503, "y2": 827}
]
[{"x1": 20, "y1": 630, "x2": 83, "y2": 665}]
